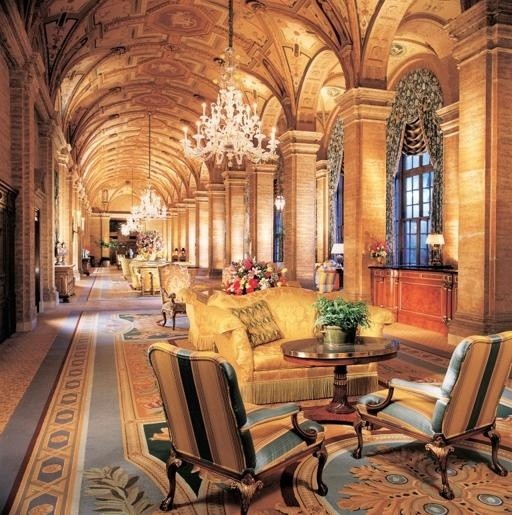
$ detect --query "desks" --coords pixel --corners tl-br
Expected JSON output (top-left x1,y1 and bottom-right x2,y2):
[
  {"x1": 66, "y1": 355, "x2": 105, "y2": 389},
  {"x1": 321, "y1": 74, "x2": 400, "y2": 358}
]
[
  {"x1": 54, "y1": 265, "x2": 77, "y2": 303},
  {"x1": 281, "y1": 336, "x2": 399, "y2": 414},
  {"x1": 81, "y1": 257, "x2": 90, "y2": 276}
]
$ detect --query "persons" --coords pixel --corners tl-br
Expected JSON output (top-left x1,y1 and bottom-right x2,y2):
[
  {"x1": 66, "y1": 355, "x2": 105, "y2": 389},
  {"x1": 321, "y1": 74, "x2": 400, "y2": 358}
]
[
  {"x1": 172, "y1": 247, "x2": 179, "y2": 262},
  {"x1": 179, "y1": 248, "x2": 186, "y2": 261}
]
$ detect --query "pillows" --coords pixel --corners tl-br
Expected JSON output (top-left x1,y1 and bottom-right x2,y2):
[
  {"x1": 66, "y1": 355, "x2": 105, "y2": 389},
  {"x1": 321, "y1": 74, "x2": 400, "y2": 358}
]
[{"x1": 228, "y1": 299, "x2": 282, "y2": 349}]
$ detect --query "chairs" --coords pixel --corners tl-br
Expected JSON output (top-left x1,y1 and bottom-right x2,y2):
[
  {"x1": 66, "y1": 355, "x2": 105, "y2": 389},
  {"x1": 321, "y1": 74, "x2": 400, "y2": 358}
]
[
  {"x1": 145, "y1": 342, "x2": 328, "y2": 514},
  {"x1": 352, "y1": 330, "x2": 511, "y2": 499},
  {"x1": 156, "y1": 263, "x2": 209, "y2": 330},
  {"x1": 116, "y1": 254, "x2": 166, "y2": 296}
]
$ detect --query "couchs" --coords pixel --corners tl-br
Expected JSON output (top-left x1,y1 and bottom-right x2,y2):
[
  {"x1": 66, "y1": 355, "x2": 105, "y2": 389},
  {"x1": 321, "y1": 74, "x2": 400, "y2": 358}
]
[
  {"x1": 179, "y1": 287, "x2": 217, "y2": 351},
  {"x1": 207, "y1": 281, "x2": 395, "y2": 404}
]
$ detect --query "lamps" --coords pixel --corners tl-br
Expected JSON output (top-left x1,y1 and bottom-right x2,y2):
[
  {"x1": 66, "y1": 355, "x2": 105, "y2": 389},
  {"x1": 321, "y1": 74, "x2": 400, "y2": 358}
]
[
  {"x1": 330, "y1": 244, "x2": 345, "y2": 266},
  {"x1": 180, "y1": 0, "x2": 281, "y2": 170},
  {"x1": 130, "y1": 109, "x2": 169, "y2": 218},
  {"x1": 119, "y1": 168, "x2": 146, "y2": 237},
  {"x1": 424, "y1": 234, "x2": 447, "y2": 266}
]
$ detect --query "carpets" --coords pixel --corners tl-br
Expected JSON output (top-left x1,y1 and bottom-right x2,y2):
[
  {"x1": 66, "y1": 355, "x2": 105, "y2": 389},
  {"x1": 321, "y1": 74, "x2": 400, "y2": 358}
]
[
  {"x1": 77, "y1": 275, "x2": 224, "y2": 302},
  {"x1": 92, "y1": 265, "x2": 122, "y2": 274},
  {"x1": 2, "y1": 286, "x2": 511, "y2": 515}
]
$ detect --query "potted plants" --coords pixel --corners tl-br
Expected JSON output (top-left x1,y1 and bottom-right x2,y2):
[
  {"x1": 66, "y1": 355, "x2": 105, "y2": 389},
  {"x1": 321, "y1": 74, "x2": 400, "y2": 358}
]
[
  {"x1": 96, "y1": 239, "x2": 123, "y2": 267},
  {"x1": 312, "y1": 295, "x2": 371, "y2": 346}
]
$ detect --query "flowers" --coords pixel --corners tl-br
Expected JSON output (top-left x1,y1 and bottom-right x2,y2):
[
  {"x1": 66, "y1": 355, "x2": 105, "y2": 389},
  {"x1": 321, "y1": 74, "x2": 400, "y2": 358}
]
[
  {"x1": 361, "y1": 231, "x2": 396, "y2": 260},
  {"x1": 222, "y1": 256, "x2": 289, "y2": 295},
  {"x1": 134, "y1": 231, "x2": 164, "y2": 254}
]
